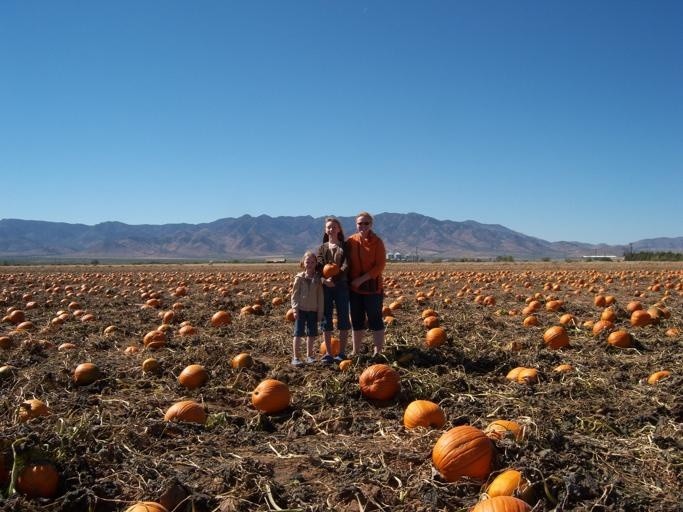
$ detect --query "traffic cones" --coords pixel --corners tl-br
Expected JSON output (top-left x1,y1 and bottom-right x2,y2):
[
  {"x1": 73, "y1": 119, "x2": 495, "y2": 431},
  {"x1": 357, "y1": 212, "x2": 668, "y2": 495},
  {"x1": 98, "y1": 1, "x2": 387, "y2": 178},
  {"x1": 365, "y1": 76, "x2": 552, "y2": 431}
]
[
  {"x1": 584, "y1": 321, "x2": 594, "y2": 328},
  {"x1": 432, "y1": 426, "x2": 494, "y2": 481},
  {"x1": 158, "y1": 325, "x2": 170, "y2": 332},
  {"x1": 7, "y1": 307, "x2": 15, "y2": 312},
  {"x1": 233, "y1": 353, "x2": 251, "y2": 367},
  {"x1": 145, "y1": 300, "x2": 158, "y2": 308},
  {"x1": 126, "y1": 502, "x2": 167, "y2": 512},
  {"x1": 383, "y1": 306, "x2": 391, "y2": 315},
  {"x1": 142, "y1": 330, "x2": 165, "y2": 347},
  {"x1": 472, "y1": 496, "x2": 532, "y2": 512},
  {"x1": 16, "y1": 322, "x2": 34, "y2": 331},
  {"x1": 424, "y1": 316, "x2": 439, "y2": 327},
  {"x1": 26, "y1": 302, "x2": 37, "y2": 308},
  {"x1": 0, "y1": 269, "x2": 295, "y2": 306},
  {"x1": 601, "y1": 310, "x2": 614, "y2": 321},
  {"x1": 60, "y1": 314, "x2": 68, "y2": 321},
  {"x1": 385, "y1": 317, "x2": 394, "y2": 322},
  {"x1": 2, "y1": 315, "x2": 10, "y2": 321},
  {"x1": 544, "y1": 326, "x2": 568, "y2": 347},
  {"x1": 389, "y1": 302, "x2": 401, "y2": 309},
  {"x1": 16, "y1": 399, "x2": 46, "y2": 420},
  {"x1": 286, "y1": 308, "x2": 295, "y2": 320},
  {"x1": 645, "y1": 307, "x2": 662, "y2": 323},
  {"x1": 76, "y1": 363, "x2": 99, "y2": 384},
  {"x1": 486, "y1": 420, "x2": 523, "y2": 442},
  {"x1": 319, "y1": 339, "x2": 341, "y2": 353},
  {"x1": 17, "y1": 466, "x2": 58, "y2": 499},
  {"x1": 179, "y1": 326, "x2": 194, "y2": 336},
  {"x1": 427, "y1": 327, "x2": 447, "y2": 346},
  {"x1": 165, "y1": 400, "x2": 204, "y2": 422},
  {"x1": 323, "y1": 263, "x2": 338, "y2": 278},
  {"x1": 530, "y1": 301, "x2": 540, "y2": 308},
  {"x1": 142, "y1": 358, "x2": 158, "y2": 373},
  {"x1": 422, "y1": 310, "x2": 438, "y2": 319},
  {"x1": 518, "y1": 368, "x2": 539, "y2": 382},
  {"x1": 0, "y1": 336, "x2": 12, "y2": 348},
  {"x1": 610, "y1": 332, "x2": 630, "y2": 346},
  {"x1": 81, "y1": 314, "x2": 96, "y2": 323},
  {"x1": 524, "y1": 306, "x2": 535, "y2": 315},
  {"x1": 163, "y1": 310, "x2": 176, "y2": 324},
  {"x1": 594, "y1": 320, "x2": 614, "y2": 332},
  {"x1": 647, "y1": 371, "x2": 671, "y2": 383},
  {"x1": 212, "y1": 311, "x2": 231, "y2": 324},
  {"x1": 665, "y1": 328, "x2": 681, "y2": 337},
  {"x1": 554, "y1": 364, "x2": 575, "y2": 372},
  {"x1": 632, "y1": 311, "x2": 648, "y2": 328},
  {"x1": 659, "y1": 308, "x2": 670, "y2": 319},
  {"x1": 178, "y1": 365, "x2": 207, "y2": 388},
  {"x1": 359, "y1": 364, "x2": 400, "y2": 400},
  {"x1": 560, "y1": 314, "x2": 576, "y2": 325},
  {"x1": 183, "y1": 326, "x2": 183, "y2": 327},
  {"x1": 82, "y1": 314, "x2": 96, "y2": 322},
  {"x1": 524, "y1": 316, "x2": 537, "y2": 326},
  {"x1": 126, "y1": 346, "x2": 139, "y2": 352},
  {"x1": 595, "y1": 296, "x2": 615, "y2": 305},
  {"x1": 1, "y1": 366, "x2": 17, "y2": 376},
  {"x1": 58, "y1": 343, "x2": 74, "y2": 349},
  {"x1": 404, "y1": 400, "x2": 446, "y2": 428},
  {"x1": 507, "y1": 368, "x2": 529, "y2": 382},
  {"x1": 252, "y1": 380, "x2": 290, "y2": 412},
  {"x1": 489, "y1": 470, "x2": 532, "y2": 499},
  {"x1": 546, "y1": 302, "x2": 560, "y2": 312},
  {"x1": 339, "y1": 360, "x2": 353, "y2": 370},
  {"x1": 10, "y1": 309, "x2": 25, "y2": 321},
  {"x1": 69, "y1": 301, "x2": 82, "y2": 309},
  {"x1": 52, "y1": 318, "x2": 62, "y2": 324},
  {"x1": 74, "y1": 310, "x2": 85, "y2": 315},
  {"x1": 104, "y1": 326, "x2": 117, "y2": 334},
  {"x1": 241, "y1": 307, "x2": 255, "y2": 317},
  {"x1": 654, "y1": 301, "x2": 665, "y2": 308},
  {"x1": 627, "y1": 303, "x2": 642, "y2": 312}
]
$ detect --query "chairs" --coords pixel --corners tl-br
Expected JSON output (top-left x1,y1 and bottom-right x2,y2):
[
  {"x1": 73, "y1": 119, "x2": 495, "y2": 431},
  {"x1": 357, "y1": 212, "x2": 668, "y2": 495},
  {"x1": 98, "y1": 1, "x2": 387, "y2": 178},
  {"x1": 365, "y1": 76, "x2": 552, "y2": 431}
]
[
  {"x1": 333, "y1": 353, "x2": 344, "y2": 361},
  {"x1": 290, "y1": 358, "x2": 303, "y2": 365},
  {"x1": 321, "y1": 354, "x2": 332, "y2": 361},
  {"x1": 305, "y1": 356, "x2": 315, "y2": 364}
]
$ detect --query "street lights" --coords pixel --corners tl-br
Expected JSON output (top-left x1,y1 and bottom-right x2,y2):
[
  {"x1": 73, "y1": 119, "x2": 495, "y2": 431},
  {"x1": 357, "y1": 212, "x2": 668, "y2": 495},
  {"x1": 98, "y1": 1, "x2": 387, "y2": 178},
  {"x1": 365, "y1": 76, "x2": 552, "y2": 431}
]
[{"x1": 359, "y1": 274, "x2": 378, "y2": 291}]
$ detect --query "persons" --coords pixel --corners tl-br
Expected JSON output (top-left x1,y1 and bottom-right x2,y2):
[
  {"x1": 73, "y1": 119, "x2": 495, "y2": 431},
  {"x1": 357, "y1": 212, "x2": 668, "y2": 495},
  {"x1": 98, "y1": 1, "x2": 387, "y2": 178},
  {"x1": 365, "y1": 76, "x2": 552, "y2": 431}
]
[
  {"x1": 316, "y1": 218, "x2": 351, "y2": 363},
  {"x1": 290, "y1": 252, "x2": 324, "y2": 367},
  {"x1": 343, "y1": 212, "x2": 387, "y2": 358}
]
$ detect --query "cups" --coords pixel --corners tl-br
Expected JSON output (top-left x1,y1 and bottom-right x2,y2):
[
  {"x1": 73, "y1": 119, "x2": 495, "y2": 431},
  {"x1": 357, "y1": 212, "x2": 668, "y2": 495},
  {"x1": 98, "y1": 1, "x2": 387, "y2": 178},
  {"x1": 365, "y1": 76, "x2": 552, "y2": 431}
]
[{"x1": 355, "y1": 221, "x2": 371, "y2": 226}]
